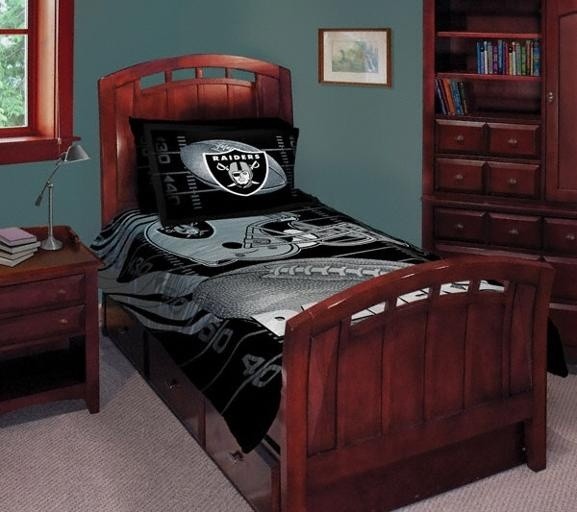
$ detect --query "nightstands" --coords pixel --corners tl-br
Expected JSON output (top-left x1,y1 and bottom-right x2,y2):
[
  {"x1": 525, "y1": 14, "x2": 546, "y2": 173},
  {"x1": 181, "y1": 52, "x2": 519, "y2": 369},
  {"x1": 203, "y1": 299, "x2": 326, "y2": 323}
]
[{"x1": 0, "y1": 225, "x2": 101, "y2": 414}]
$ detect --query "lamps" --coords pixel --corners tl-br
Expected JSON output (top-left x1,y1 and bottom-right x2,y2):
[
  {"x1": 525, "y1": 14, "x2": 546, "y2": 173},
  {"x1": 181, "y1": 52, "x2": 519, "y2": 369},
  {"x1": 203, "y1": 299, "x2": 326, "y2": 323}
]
[{"x1": 34, "y1": 143, "x2": 91, "y2": 251}]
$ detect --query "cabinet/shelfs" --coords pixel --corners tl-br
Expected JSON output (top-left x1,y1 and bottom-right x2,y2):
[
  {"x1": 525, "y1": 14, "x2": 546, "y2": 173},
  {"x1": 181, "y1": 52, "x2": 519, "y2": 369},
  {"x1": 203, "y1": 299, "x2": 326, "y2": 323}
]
[{"x1": 421, "y1": 0, "x2": 577, "y2": 365}]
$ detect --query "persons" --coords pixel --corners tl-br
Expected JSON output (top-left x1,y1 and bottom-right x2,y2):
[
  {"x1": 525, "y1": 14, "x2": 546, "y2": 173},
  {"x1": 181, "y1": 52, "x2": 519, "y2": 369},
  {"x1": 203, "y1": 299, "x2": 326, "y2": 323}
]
[{"x1": 228, "y1": 161, "x2": 253, "y2": 188}]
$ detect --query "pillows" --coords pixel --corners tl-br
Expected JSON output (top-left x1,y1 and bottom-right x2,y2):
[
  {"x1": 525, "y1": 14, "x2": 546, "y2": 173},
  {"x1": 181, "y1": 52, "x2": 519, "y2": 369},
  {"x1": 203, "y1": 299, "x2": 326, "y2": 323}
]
[
  {"x1": 142, "y1": 124, "x2": 300, "y2": 227},
  {"x1": 127, "y1": 114, "x2": 283, "y2": 215}
]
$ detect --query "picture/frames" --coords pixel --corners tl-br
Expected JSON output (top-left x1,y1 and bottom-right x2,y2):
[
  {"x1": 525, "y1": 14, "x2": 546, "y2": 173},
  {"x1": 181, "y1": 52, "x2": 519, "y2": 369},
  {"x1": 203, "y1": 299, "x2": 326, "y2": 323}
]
[{"x1": 318, "y1": 28, "x2": 392, "y2": 86}]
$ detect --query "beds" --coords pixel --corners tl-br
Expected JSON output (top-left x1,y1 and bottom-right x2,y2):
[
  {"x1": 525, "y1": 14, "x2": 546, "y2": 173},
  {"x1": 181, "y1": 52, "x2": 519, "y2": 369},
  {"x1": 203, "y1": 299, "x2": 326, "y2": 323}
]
[{"x1": 99, "y1": 54, "x2": 554, "y2": 512}]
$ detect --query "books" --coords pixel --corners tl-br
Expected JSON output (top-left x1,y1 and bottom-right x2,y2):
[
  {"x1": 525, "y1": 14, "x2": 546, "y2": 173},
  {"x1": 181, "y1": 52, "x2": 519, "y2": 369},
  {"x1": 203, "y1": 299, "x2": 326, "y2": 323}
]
[
  {"x1": 435, "y1": 78, "x2": 468, "y2": 116},
  {"x1": 477, "y1": 39, "x2": 539, "y2": 76},
  {"x1": 0, "y1": 227, "x2": 41, "y2": 267}
]
